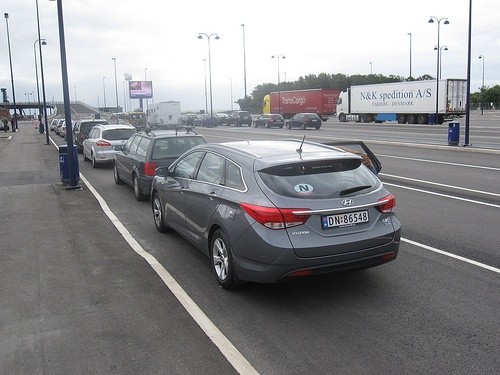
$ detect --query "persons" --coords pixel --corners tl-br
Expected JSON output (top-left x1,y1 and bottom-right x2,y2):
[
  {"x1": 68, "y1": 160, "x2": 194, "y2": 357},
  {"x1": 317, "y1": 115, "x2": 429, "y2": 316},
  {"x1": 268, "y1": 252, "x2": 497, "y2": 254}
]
[
  {"x1": 11, "y1": 118, "x2": 15, "y2": 132},
  {"x1": 2, "y1": 116, "x2": 8, "y2": 132}
]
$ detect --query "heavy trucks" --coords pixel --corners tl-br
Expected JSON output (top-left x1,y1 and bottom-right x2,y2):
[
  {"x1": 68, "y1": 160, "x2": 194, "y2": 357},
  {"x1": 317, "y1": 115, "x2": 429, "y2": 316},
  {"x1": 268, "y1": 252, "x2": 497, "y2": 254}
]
[
  {"x1": 335, "y1": 78, "x2": 467, "y2": 125},
  {"x1": 263, "y1": 88, "x2": 340, "y2": 122}
]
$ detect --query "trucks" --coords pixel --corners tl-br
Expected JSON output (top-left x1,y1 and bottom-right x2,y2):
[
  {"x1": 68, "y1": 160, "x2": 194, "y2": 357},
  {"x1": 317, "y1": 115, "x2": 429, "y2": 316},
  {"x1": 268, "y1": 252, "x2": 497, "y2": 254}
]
[{"x1": 147, "y1": 101, "x2": 180, "y2": 130}]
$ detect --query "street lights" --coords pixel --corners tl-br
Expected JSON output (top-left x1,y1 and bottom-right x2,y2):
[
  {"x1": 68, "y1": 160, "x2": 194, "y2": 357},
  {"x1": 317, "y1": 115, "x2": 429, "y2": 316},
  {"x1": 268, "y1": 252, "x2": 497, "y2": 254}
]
[
  {"x1": 428, "y1": 14, "x2": 450, "y2": 125},
  {"x1": 478, "y1": 54, "x2": 485, "y2": 115},
  {"x1": 434, "y1": 44, "x2": 449, "y2": 80},
  {"x1": 103, "y1": 76, "x2": 106, "y2": 107},
  {"x1": 241, "y1": 23, "x2": 245, "y2": 97},
  {"x1": 34, "y1": 38, "x2": 48, "y2": 133},
  {"x1": 144, "y1": 68, "x2": 148, "y2": 115},
  {"x1": 4, "y1": 12, "x2": 18, "y2": 128},
  {"x1": 408, "y1": 33, "x2": 412, "y2": 78},
  {"x1": 112, "y1": 57, "x2": 119, "y2": 112},
  {"x1": 202, "y1": 58, "x2": 207, "y2": 113},
  {"x1": 370, "y1": 61, "x2": 372, "y2": 75},
  {"x1": 272, "y1": 53, "x2": 285, "y2": 113},
  {"x1": 25, "y1": 92, "x2": 32, "y2": 121},
  {"x1": 198, "y1": 32, "x2": 220, "y2": 127}
]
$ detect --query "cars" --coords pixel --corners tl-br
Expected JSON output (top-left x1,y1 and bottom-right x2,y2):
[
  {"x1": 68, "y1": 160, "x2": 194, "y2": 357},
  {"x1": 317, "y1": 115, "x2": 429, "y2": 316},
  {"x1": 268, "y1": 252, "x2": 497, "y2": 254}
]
[{"x1": 180, "y1": 111, "x2": 322, "y2": 130}]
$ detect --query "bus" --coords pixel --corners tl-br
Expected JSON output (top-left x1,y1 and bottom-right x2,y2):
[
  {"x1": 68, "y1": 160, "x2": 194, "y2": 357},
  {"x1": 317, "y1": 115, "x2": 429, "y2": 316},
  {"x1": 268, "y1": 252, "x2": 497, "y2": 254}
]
[
  {"x1": 128, "y1": 112, "x2": 147, "y2": 130},
  {"x1": 110, "y1": 112, "x2": 130, "y2": 125}
]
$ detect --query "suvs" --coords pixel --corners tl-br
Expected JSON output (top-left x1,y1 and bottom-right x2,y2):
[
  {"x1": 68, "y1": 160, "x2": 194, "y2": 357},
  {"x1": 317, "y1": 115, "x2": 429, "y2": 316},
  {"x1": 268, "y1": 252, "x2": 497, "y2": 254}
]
[
  {"x1": 113, "y1": 125, "x2": 210, "y2": 201},
  {"x1": 80, "y1": 122, "x2": 138, "y2": 168},
  {"x1": 47, "y1": 118, "x2": 112, "y2": 155},
  {"x1": 150, "y1": 138, "x2": 402, "y2": 291}
]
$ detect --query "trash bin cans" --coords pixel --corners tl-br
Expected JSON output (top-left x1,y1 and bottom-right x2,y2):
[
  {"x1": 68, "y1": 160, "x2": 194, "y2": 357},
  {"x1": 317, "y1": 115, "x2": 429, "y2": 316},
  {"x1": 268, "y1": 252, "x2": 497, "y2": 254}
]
[
  {"x1": 40, "y1": 123, "x2": 44, "y2": 133},
  {"x1": 448, "y1": 123, "x2": 460, "y2": 144},
  {"x1": 59, "y1": 145, "x2": 80, "y2": 182},
  {"x1": 429, "y1": 113, "x2": 436, "y2": 125}
]
